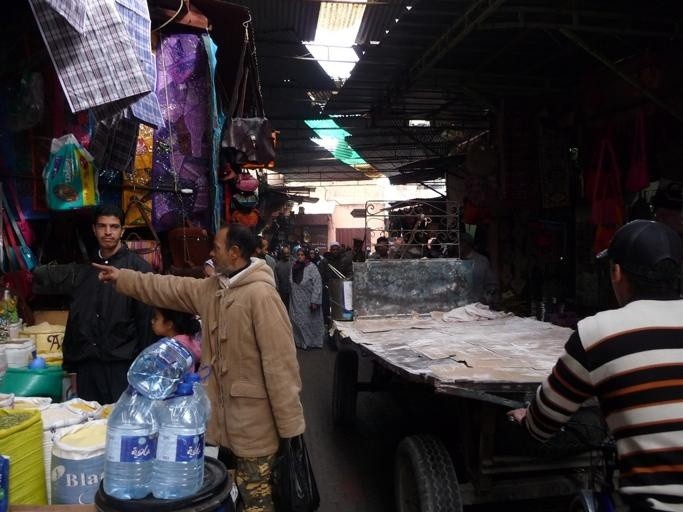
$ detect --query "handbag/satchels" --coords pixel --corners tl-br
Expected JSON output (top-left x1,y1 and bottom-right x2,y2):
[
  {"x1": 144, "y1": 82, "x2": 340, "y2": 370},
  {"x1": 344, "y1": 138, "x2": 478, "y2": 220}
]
[
  {"x1": 1, "y1": 168, "x2": 36, "y2": 328},
  {"x1": 118, "y1": 200, "x2": 164, "y2": 272},
  {"x1": 219, "y1": 24, "x2": 281, "y2": 226},
  {"x1": 269, "y1": 432, "x2": 321, "y2": 512},
  {"x1": 169, "y1": 220, "x2": 209, "y2": 269}
]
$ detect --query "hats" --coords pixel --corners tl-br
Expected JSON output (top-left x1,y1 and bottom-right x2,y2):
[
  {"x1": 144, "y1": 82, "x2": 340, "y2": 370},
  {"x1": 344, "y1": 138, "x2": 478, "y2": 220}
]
[{"x1": 595, "y1": 219, "x2": 681, "y2": 282}]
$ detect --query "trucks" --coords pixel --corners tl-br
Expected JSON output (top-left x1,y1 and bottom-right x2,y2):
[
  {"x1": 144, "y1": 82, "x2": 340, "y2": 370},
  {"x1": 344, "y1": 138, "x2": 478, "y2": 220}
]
[{"x1": 324, "y1": 254, "x2": 620, "y2": 511}]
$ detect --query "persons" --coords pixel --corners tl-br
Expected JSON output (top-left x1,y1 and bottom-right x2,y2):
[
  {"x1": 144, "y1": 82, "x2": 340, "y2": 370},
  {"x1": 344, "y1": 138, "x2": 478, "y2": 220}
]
[
  {"x1": 151, "y1": 307, "x2": 202, "y2": 371},
  {"x1": 505, "y1": 218, "x2": 683, "y2": 512},
  {"x1": 198, "y1": 226, "x2": 496, "y2": 352},
  {"x1": 91, "y1": 223, "x2": 306, "y2": 512},
  {"x1": 61, "y1": 202, "x2": 156, "y2": 405}
]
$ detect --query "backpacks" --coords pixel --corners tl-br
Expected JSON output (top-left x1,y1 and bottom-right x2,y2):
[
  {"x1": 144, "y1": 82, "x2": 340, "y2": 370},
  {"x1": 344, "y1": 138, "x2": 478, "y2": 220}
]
[{"x1": 43, "y1": 144, "x2": 101, "y2": 212}]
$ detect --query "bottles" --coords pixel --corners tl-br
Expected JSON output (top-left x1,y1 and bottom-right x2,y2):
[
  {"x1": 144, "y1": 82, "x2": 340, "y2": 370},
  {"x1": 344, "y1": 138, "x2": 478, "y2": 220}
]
[
  {"x1": 150, "y1": 370, "x2": 210, "y2": 500},
  {"x1": 126, "y1": 335, "x2": 195, "y2": 402},
  {"x1": 102, "y1": 386, "x2": 157, "y2": 500}
]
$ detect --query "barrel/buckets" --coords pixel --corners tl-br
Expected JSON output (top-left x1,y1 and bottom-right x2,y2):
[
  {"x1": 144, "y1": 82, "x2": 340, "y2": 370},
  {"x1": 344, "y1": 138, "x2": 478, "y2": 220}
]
[
  {"x1": 95, "y1": 454, "x2": 242, "y2": 512},
  {"x1": 328, "y1": 278, "x2": 354, "y2": 321},
  {"x1": 103, "y1": 336, "x2": 212, "y2": 499}
]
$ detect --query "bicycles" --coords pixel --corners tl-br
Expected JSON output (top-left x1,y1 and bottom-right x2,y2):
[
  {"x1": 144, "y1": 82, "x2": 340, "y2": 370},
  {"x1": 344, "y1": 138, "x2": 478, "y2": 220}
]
[{"x1": 507, "y1": 414, "x2": 619, "y2": 512}]
